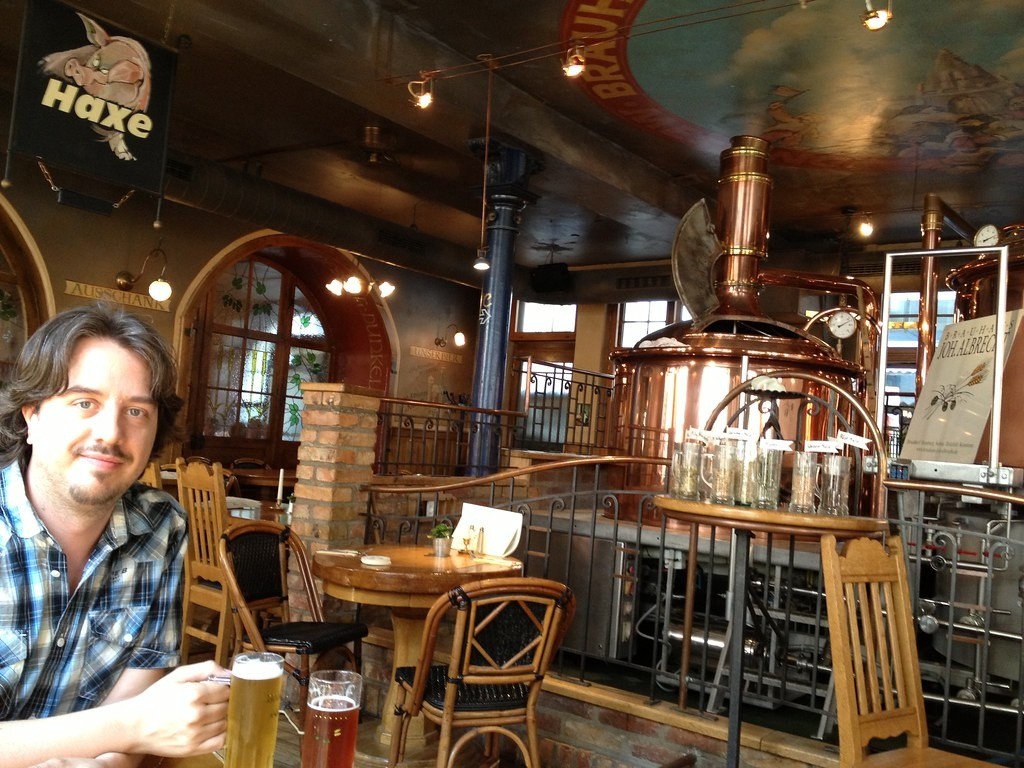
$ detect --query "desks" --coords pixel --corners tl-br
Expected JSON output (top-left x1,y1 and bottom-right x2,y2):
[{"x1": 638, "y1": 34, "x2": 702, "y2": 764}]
[
  {"x1": 653, "y1": 493, "x2": 890, "y2": 768},
  {"x1": 313, "y1": 544, "x2": 523, "y2": 768},
  {"x1": 230, "y1": 469, "x2": 296, "y2": 487},
  {"x1": 193, "y1": 496, "x2": 292, "y2": 527}
]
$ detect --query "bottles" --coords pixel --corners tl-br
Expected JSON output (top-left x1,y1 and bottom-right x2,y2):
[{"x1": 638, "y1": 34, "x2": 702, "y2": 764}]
[
  {"x1": 477, "y1": 527, "x2": 484, "y2": 554},
  {"x1": 467, "y1": 524, "x2": 476, "y2": 551}
]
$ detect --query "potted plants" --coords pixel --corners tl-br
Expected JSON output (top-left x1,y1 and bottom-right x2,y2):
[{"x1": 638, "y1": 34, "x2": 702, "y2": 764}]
[{"x1": 429, "y1": 524, "x2": 453, "y2": 556}]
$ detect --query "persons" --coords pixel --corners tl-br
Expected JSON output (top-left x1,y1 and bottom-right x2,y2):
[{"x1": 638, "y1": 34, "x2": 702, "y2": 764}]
[{"x1": 0, "y1": 306, "x2": 230, "y2": 768}]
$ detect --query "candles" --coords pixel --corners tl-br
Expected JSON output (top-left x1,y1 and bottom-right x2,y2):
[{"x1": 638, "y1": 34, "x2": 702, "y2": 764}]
[{"x1": 277, "y1": 468, "x2": 284, "y2": 499}]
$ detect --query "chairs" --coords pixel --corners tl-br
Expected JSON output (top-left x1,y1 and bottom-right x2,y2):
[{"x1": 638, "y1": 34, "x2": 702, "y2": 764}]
[
  {"x1": 136, "y1": 456, "x2": 271, "y2": 667},
  {"x1": 219, "y1": 521, "x2": 369, "y2": 756},
  {"x1": 388, "y1": 577, "x2": 576, "y2": 768},
  {"x1": 821, "y1": 534, "x2": 1010, "y2": 768}
]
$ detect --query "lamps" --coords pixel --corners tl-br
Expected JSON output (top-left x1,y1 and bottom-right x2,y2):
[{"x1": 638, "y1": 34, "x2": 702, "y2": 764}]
[
  {"x1": 473, "y1": 249, "x2": 490, "y2": 270},
  {"x1": 406, "y1": 78, "x2": 434, "y2": 109},
  {"x1": 562, "y1": 46, "x2": 587, "y2": 76},
  {"x1": 856, "y1": 211, "x2": 874, "y2": 239},
  {"x1": 326, "y1": 255, "x2": 396, "y2": 298},
  {"x1": 435, "y1": 324, "x2": 465, "y2": 347},
  {"x1": 857, "y1": 0, "x2": 893, "y2": 31},
  {"x1": 117, "y1": 248, "x2": 172, "y2": 301}
]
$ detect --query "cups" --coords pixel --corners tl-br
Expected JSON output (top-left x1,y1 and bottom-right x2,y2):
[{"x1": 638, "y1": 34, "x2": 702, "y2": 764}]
[
  {"x1": 788, "y1": 451, "x2": 820, "y2": 515},
  {"x1": 207, "y1": 652, "x2": 285, "y2": 768},
  {"x1": 300, "y1": 670, "x2": 362, "y2": 768},
  {"x1": 673, "y1": 432, "x2": 757, "y2": 509},
  {"x1": 754, "y1": 448, "x2": 782, "y2": 511},
  {"x1": 816, "y1": 455, "x2": 852, "y2": 517}
]
